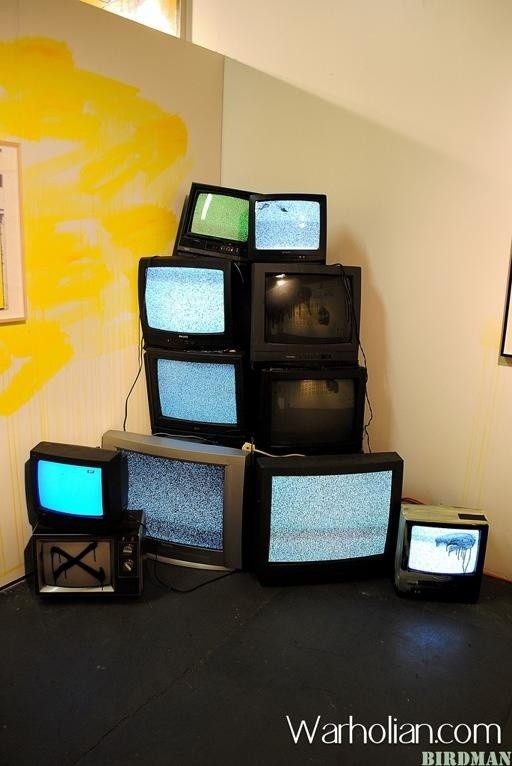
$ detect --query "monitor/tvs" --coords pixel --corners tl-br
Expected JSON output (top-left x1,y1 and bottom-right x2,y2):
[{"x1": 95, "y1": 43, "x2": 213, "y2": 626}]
[
  {"x1": 396, "y1": 503, "x2": 490, "y2": 603},
  {"x1": 25, "y1": 508, "x2": 148, "y2": 603},
  {"x1": 249, "y1": 193, "x2": 327, "y2": 264},
  {"x1": 138, "y1": 257, "x2": 242, "y2": 353},
  {"x1": 24, "y1": 441, "x2": 129, "y2": 527},
  {"x1": 245, "y1": 366, "x2": 367, "y2": 456},
  {"x1": 143, "y1": 349, "x2": 246, "y2": 450},
  {"x1": 242, "y1": 450, "x2": 404, "y2": 595},
  {"x1": 102, "y1": 430, "x2": 248, "y2": 575},
  {"x1": 176, "y1": 181, "x2": 255, "y2": 261},
  {"x1": 250, "y1": 261, "x2": 361, "y2": 364}
]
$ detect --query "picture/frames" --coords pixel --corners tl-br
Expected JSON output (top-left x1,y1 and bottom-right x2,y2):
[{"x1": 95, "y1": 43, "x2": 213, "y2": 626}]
[{"x1": 0, "y1": 139, "x2": 27, "y2": 324}]
